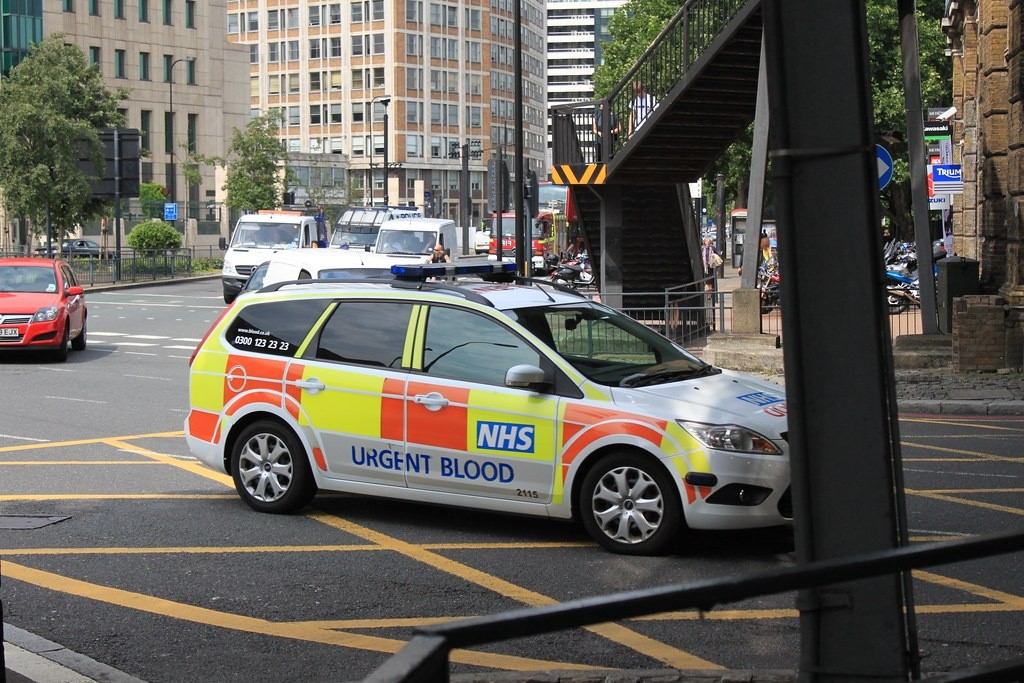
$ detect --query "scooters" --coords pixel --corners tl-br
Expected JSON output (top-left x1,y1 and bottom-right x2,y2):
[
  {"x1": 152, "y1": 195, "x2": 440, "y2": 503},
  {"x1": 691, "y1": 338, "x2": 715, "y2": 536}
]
[{"x1": 550, "y1": 263, "x2": 598, "y2": 293}]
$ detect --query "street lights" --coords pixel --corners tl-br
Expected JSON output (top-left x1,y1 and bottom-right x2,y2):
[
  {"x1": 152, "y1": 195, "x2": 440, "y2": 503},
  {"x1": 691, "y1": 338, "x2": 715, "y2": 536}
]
[
  {"x1": 367, "y1": 94, "x2": 390, "y2": 206},
  {"x1": 169, "y1": 55, "x2": 195, "y2": 227}
]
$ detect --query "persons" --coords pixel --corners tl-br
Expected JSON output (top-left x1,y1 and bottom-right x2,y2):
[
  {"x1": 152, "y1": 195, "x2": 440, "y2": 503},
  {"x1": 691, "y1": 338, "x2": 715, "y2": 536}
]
[
  {"x1": 700, "y1": 236, "x2": 717, "y2": 300},
  {"x1": 628, "y1": 81, "x2": 659, "y2": 141},
  {"x1": 425, "y1": 244, "x2": 453, "y2": 281},
  {"x1": 384, "y1": 235, "x2": 402, "y2": 252},
  {"x1": 593, "y1": 96, "x2": 622, "y2": 163},
  {"x1": 422, "y1": 236, "x2": 437, "y2": 254}
]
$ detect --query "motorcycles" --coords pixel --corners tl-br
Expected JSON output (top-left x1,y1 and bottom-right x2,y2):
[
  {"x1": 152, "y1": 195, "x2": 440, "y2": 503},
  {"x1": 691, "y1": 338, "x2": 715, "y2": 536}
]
[{"x1": 755, "y1": 238, "x2": 950, "y2": 315}]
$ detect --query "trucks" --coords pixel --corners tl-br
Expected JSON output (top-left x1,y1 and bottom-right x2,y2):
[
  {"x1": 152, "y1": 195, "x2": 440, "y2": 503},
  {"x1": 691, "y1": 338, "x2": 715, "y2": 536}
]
[
  {"x1": 524, "y1": 179, "x2": 585, "y2": 260},
  {"x1": 489, "y1": 210, "x2": 558, "y2": 258}
]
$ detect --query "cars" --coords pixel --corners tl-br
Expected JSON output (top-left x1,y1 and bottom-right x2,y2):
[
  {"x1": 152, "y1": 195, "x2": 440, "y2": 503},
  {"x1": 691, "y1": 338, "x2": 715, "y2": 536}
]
[
  {"x1": 182, "y1": 256, "x2": 799, "y2": 554},
  {"x1": 0, "y1": 257, "x2": 89, "y2": 363},
  {"x1": 33, "y1": 239, "x2": 116, "y2": 259}
]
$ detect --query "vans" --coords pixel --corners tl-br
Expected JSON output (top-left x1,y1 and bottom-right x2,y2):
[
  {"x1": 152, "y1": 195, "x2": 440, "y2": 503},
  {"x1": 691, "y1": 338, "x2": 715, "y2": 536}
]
[
  {"x1": 329, "y1": 205, "x2": 418, "y2": 252},
  {"x1": 374, "y1": 218, "x2": 457, "y2": 264},
  {"x1": 218, "y1": 209, "x2": 318, "y2": 304}
]
